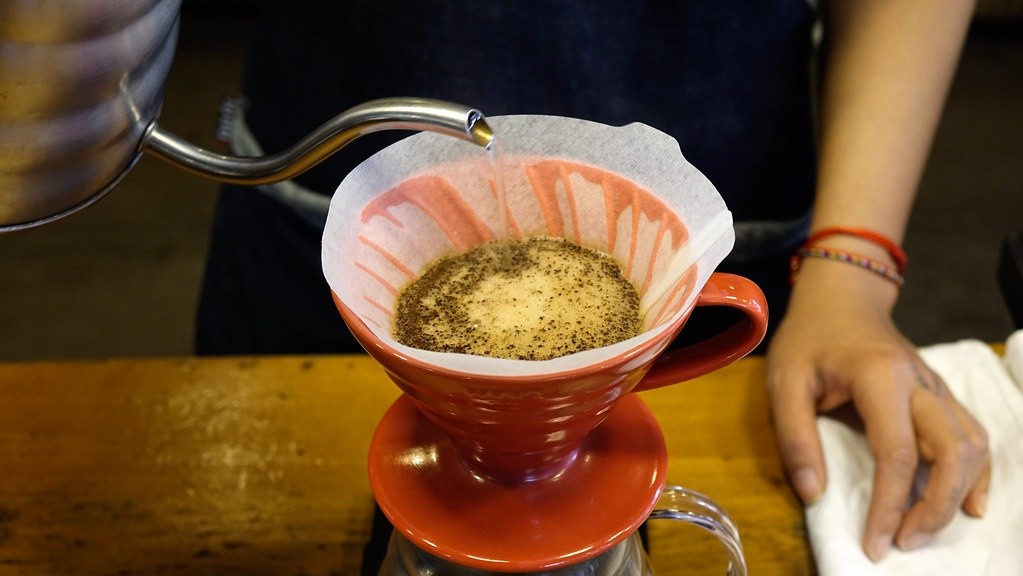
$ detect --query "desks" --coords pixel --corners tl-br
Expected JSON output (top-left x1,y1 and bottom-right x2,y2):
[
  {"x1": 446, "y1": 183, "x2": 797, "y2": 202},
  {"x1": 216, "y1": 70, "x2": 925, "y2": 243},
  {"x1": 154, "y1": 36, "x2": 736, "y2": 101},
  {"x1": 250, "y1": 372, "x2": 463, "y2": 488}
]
[{"x1": 0, "y1": 340, "x2": 1006, "y2": 576}]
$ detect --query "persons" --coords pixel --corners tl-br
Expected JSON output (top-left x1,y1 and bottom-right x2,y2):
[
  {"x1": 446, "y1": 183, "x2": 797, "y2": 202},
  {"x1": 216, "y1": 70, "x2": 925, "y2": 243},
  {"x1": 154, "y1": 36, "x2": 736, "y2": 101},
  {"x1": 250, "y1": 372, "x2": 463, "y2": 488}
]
[{"x1": 194, "y1": 0, "x2": 1023, "y2": 564}]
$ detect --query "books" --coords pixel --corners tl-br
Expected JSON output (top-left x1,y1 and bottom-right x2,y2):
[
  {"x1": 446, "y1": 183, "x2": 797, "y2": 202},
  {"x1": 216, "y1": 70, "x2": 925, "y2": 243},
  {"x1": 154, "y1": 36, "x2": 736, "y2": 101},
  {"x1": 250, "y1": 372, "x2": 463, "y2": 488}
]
[{"x1": 804, "y1": 330, "x2": 1023, "y2": 576}]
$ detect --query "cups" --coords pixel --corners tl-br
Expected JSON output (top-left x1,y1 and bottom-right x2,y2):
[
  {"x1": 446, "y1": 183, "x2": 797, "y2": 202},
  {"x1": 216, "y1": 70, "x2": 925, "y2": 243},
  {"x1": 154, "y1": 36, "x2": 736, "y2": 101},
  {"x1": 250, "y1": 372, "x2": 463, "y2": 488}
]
[
  {"x1": 319, "y1": 113, "x2": 770, "y2": 485},
  {"x1": 378, "y1": 486, "x2": 750, "y2": 576}
]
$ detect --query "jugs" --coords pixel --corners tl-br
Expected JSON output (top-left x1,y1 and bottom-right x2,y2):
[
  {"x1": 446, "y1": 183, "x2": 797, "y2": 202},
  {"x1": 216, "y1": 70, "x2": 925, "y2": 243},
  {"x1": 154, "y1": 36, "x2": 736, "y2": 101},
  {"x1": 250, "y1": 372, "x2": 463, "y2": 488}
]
[{"x1": 0, "y1": 0, "x2": 493, "y2": 233}]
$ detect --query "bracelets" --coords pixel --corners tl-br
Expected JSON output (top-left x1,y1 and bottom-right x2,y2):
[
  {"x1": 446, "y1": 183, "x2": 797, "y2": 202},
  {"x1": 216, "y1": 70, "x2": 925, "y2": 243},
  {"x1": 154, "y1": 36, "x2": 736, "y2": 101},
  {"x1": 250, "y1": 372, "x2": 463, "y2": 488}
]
[
  {"x1": 805, "y1": 227, "x2": 907, "y2": 273},
  {"x1": 791, "y1": 247, "x2": 904, "y2": 288}
]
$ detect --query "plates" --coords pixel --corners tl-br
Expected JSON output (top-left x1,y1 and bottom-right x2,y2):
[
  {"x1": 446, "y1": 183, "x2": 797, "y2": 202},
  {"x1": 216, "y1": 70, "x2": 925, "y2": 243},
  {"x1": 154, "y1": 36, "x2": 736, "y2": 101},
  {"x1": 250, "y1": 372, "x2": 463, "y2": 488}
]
[{"x1": 368, "y1": 391, "x2": 669, "y2": 572}]
